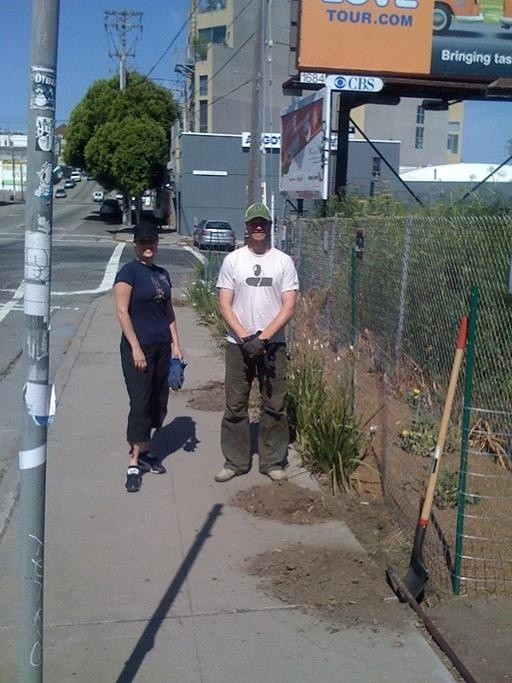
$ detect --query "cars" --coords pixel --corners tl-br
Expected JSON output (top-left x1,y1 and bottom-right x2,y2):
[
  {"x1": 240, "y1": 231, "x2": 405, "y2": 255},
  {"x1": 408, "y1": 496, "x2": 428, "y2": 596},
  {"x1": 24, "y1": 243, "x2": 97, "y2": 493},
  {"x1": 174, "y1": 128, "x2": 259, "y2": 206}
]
[
  {"x1": 54, "y1": 165, "x2": 124, "y2": 219},
  {"x1": 432, "y1": 0, "x2": 484, "y2": 34},
  {"x1": 193, "y1": 218, "x2": 236, "y2": 251}
]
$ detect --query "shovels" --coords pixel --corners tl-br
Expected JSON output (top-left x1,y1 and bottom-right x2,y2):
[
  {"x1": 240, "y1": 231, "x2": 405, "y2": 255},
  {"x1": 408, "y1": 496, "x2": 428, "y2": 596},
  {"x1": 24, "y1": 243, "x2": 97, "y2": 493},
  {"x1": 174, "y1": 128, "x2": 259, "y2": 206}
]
[{"x1": 401, "y1": 317, "x2": 468, "y2": 601}]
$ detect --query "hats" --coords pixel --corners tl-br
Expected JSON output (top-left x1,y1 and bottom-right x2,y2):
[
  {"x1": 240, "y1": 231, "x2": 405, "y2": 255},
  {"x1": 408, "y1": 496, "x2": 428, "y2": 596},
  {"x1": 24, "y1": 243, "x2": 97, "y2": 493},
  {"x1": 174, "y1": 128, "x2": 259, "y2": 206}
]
[
  {"x1": 244, "y1": 202, "x2": 271, "y2": 223},
  {"x1": 134, "y1": 221, "x2": 159, "y2": 242}
]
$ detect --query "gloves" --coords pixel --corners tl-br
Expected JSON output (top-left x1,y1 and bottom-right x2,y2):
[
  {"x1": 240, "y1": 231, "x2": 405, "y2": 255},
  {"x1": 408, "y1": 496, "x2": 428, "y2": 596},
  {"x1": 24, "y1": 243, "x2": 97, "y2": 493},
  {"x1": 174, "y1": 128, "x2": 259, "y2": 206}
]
[
  {"x1": 168, "y1": 359, "x2": 188, "y2": 390},
  {"x1": 241, "y1": 330, "x2": 267, "y2": 359}
]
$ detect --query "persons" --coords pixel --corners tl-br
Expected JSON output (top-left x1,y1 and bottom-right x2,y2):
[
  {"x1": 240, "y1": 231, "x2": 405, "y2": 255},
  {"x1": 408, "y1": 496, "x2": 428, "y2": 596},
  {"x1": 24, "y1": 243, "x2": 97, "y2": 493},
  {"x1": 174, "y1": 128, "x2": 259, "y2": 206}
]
[
  {"x1": 214, "y1": 204, "x2": 301, "y2": 483},
  {"x1": 114, "y1": 220, "x2": 181, "y2": 493}
]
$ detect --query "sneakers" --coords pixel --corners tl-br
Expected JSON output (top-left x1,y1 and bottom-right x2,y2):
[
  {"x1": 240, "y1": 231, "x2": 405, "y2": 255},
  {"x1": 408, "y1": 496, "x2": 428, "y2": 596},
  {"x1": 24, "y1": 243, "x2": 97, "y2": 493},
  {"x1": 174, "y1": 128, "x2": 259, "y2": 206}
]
[
  {"x1": 269, "y1": 469, "x2": 288, "y2": 481},
  {"x1": 126, "y1": 467, "x2": 141, "y2": 492},
  {"x1": 138, "y1": 456, "x2": 166, "y2": 474},
  {"x1": 215, "y1": 468, "x2": 235, "y2": 481}
]
